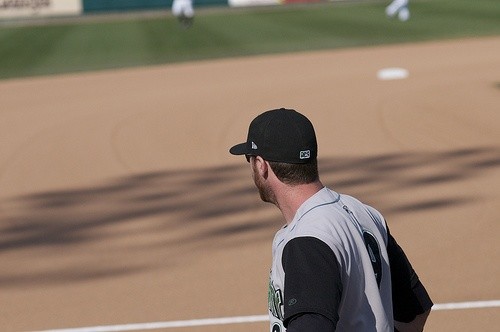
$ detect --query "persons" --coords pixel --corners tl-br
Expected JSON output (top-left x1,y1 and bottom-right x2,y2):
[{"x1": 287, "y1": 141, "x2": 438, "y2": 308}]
[
  {"x1": 228, "y1": 108, "x2": 437, "y2": 332},
  {"x1": 171, "y1": 0, "x2": 197, "y2": 28},
  {"x1": 384, "y1": 0, "x2": 410, "y2": 21}
]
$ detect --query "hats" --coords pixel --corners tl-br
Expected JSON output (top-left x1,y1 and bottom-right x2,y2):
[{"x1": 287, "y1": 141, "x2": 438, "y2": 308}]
[{"x1": 229, "y1": 108, "x2": 318, "y2": 164}]
